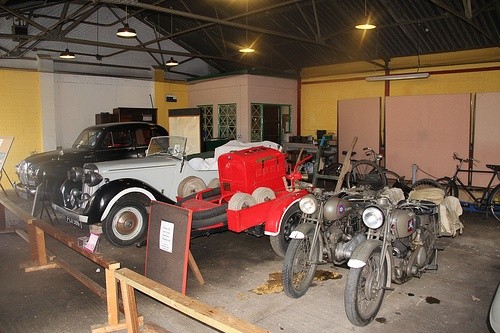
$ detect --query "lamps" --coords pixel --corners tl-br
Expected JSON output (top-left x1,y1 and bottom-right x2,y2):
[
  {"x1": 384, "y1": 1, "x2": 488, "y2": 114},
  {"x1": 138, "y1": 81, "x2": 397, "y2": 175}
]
[
  {"x1": 116, "y1": 0, "x2": 137, "y2": 38},
  {"x1": 354, "y1": 1, "x2": 377, "y2": 30},
  {"x1": 238, "y1": 0, "x2": 255, "y2": 52},
  {"x1": 364, "y1": 20, "x2": 430, "y2": 81},
  {"x1": 58, "y1": 15, "x2": 76, "y2": 59},
  {"x1": 165, "y1": 11, "x2": 179, "y2": 66}
]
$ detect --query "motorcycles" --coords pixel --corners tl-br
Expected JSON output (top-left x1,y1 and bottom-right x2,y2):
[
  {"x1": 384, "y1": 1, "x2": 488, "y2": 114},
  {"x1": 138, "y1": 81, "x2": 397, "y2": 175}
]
[
  {"x1": 344, "y1": 176, "x2": 453, "y2": 327},
  {"x1": 283, "y1": 162, "x2": 389, "y2": 297}
]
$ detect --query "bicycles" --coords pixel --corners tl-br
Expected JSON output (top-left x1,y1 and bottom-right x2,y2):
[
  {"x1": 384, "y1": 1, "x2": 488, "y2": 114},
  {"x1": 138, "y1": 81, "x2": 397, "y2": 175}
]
[
  {"x1": 435, "y1": 152, "x2": 500, "y2": 223},
  {"x1": 323, "y1": 150, "x2": 403, "y2": 190}
]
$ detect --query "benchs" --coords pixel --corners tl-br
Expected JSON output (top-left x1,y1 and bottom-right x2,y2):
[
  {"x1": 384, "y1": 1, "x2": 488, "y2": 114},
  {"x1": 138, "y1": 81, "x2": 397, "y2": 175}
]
[{"x1": 213, "y1": 139, "x2": 280, "y2": 159}]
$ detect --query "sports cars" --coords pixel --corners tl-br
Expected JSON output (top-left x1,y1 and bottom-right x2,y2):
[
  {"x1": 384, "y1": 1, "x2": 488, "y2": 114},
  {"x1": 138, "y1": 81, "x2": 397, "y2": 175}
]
[{"x1": 50, "y1": 135, "x2": 283, "y2": 248}]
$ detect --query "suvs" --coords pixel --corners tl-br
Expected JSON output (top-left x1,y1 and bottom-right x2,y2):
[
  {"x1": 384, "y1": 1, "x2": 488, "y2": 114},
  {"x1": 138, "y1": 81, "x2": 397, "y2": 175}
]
[{"x1": 13, "y1": 121, "x2": 170, "y2": 203}]
